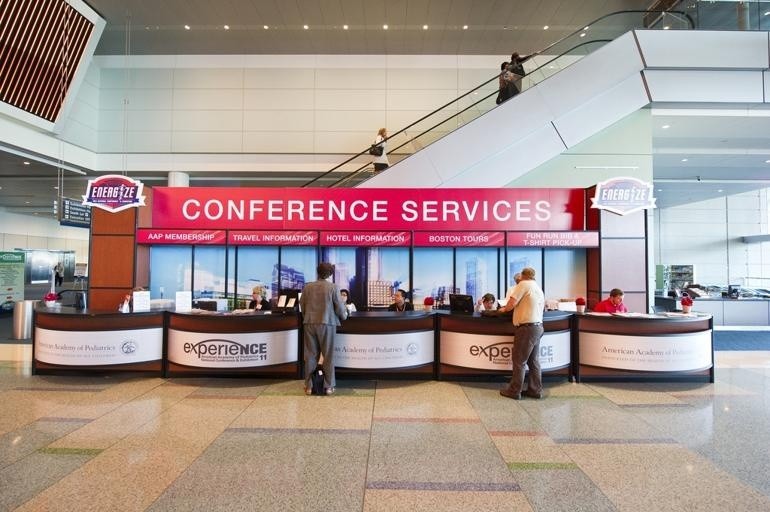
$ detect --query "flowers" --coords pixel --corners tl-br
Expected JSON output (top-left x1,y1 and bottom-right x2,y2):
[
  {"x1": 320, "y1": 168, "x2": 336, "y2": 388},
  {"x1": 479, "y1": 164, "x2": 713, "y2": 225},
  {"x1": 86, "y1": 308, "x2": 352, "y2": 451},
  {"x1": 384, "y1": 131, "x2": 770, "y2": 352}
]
[
  {"x1": 43, "y1": 294, "x2": 57, "y2": 301},
  {"x1": 424, "y1": 297, "x2": 434, "y2": 305},
  {"x1": 681, "y1": 296, "x2": 693, "y2": 306},
  {"x1": 576, "y1": 298, "x2": 586, "y2": 304}
]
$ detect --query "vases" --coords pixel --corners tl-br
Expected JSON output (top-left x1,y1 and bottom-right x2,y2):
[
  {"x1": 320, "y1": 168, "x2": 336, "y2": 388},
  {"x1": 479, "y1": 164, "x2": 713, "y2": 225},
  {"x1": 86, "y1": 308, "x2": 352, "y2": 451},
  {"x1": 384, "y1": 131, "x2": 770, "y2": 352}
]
[
  {"x1": 45, "y1": 300, "x2": 55, "y2": 306},
  {"x1": 682, "y1": 304, "x2": 691, "y2": 312},
  {"x1": 576, "y1": 304, "x2": 585, "y2": 313},
  {"x1": 425, "y1": 304, "x2": 432, "y2": 312}
]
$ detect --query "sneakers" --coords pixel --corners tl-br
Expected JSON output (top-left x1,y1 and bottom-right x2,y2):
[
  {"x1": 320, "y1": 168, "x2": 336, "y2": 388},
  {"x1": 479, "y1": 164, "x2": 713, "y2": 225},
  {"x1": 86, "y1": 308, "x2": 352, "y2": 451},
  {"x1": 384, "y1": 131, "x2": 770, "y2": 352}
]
[
  {"x1": 499, "y1": 389, "x2": 521, "y2": 400},
  {"x1": 303, "y1": 386, "x2": 312, "y2": 395},
  {"x1": 325, "y1": 387, "x2": 336, "y2": 396},
  {"x1": 521, "y1": 391, "x2": 542, "y2": 399}
]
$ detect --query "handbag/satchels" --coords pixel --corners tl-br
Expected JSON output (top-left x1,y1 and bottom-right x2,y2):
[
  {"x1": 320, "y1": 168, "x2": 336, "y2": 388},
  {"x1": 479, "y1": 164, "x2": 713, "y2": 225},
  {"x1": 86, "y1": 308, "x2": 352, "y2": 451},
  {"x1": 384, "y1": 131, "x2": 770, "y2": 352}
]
[{"x1": 311, "y1": 365, "x2": 324, "y2": 396}]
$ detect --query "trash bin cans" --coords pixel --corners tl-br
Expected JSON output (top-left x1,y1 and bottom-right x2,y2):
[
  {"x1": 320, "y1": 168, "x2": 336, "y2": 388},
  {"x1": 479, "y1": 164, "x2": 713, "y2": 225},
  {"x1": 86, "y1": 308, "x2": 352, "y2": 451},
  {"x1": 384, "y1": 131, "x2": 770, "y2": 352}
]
[{"x1": 12, "y1": 299, "x2": 41, "y2": 339}]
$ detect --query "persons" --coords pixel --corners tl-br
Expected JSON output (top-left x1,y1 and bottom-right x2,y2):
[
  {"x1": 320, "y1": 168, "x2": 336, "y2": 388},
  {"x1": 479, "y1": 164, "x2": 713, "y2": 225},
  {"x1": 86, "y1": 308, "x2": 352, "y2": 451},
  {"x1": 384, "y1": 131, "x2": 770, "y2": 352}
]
[
  {"x1": 593, "y1": 288, "x2": 628, "y2": 314},
  {"x1": 388, "y1": 289, "x2": 414, "y2": 311},
  {"x1": 249, "y1": 285, "x2": 270, "y2": 311},
  {"x1": 368, "y1": 128, "x2": 390, "y2": 174},
  {"x1": 503, "y1": 50, "x2": 542, "y2": 103},
  {"x1": 340, "y1": 289, "x2": 358, "y2": 314},
  {"x1": 299, "y1": 262, "x2": 349, "y2": 397},
  {"x1": 495, "y1": 61, "x2": 509, "y2": 105},
  {"x1": 497, "y1": 267, "x2": 544, "y2": 401},
  {"x1": 54, "y1": 261, "x2": 64, "y2": 287},
  {"x1": 474, "y1": 292, "x2": 497, "y2": 313},
  {"x1": 505, "y1": 272, "x2": 522, "y2": 299}
]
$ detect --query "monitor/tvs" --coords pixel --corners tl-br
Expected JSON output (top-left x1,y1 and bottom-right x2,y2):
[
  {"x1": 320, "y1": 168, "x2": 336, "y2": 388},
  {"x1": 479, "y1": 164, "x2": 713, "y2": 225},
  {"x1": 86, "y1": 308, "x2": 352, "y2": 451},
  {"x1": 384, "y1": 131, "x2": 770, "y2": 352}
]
[
  {"x1": 198, "y1": 301, "x2": 217, "y2": 311},
  {"x1": 655, "y1": 297, "x2": 676, "y2": 312},
  {"x1": 75, "y1": 292, "x2": 86, "y2": 308},
  {"x1": 449, "y1": 294, "x2": 474, "y2": 312}
]
